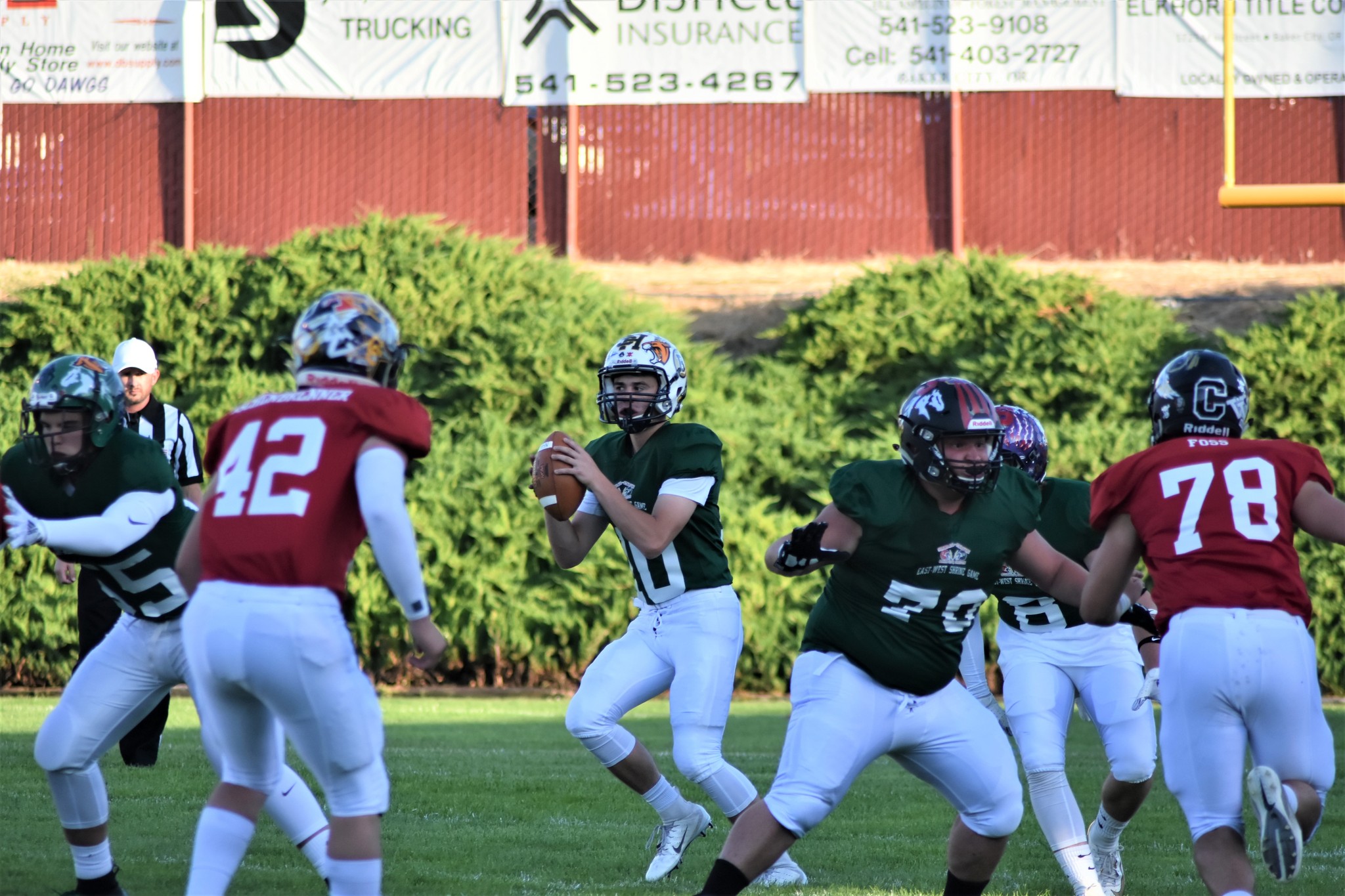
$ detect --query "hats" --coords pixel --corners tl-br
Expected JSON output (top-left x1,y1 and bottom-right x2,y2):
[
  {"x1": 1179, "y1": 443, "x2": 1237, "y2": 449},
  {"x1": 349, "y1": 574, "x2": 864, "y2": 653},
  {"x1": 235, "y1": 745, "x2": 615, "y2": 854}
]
[{"x1": 112, "y1": 337, "x2": 157, "y2": 374}]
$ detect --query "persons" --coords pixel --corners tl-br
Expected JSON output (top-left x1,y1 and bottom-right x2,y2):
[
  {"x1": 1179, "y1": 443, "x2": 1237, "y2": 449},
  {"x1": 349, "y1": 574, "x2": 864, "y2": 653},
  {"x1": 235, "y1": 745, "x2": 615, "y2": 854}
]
[
  {"x1": 1, "y1": 291, "x2": 450, "y2": 895},
  {"x1": 527, "y1": 331, "x2": 810, "y2": 889},
  {"x1": 693, "y1": 351, "x2": 1345, "y2": 896}
]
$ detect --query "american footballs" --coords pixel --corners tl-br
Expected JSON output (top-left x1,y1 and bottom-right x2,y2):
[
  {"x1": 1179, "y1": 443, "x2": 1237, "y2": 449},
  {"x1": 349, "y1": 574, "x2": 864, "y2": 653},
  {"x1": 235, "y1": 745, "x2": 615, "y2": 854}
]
[{"x1": 532, "y1": 431, "x2": 587, "y2": 521}]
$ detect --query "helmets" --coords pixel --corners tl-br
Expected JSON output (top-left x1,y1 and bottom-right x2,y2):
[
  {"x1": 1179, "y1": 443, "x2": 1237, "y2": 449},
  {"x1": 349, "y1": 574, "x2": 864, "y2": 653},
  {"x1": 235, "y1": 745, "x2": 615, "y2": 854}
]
[
  {"x1": 1147, "y1": 348, "x2": 1251, "y2": 447},
  {"x1": 292, "y1": 290, "x2": 406, "y2": 391},
  {"x1": 892, "y1": 376, "x2": 1006, "y2": 490},
  {"x1": 20, "y1": 354, "x2": 126, "y2": 477},
  {"x1": 994, "y1": 404, "x2": 1049, "y2": 485},
  {"x1": 596, "y1": 332, "x2": 687, "y2": 434}
]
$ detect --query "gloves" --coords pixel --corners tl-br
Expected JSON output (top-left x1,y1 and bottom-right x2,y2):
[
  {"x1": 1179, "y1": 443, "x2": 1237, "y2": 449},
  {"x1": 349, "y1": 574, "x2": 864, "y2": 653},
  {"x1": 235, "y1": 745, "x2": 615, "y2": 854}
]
[
  {"x1": 773, "y1": 521, "x2": 852, "y2": 572},
  {"x1": 2, "y1": 485, "x2": 46, "y2": 550},
  {"x1": 1131, "y1": 667, "x2": 1161, "y2": 711}
]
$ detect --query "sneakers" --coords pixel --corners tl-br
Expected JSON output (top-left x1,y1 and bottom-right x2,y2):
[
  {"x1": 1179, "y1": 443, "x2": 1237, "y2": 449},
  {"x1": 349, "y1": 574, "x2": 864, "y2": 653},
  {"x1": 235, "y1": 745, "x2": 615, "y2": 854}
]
[
  {"x1": 645, "y1": 803, "x2": 713, "y2": 883},
  {"x1": 1087, "y1": 819, "x2": 1125, "y2": 896},
  {"x1": 1246, "y1": 765, "x2": 1303, "y2": 882},
  {"x1": 751, "y1": 862, "x2": 808, "y2": 887}
]
